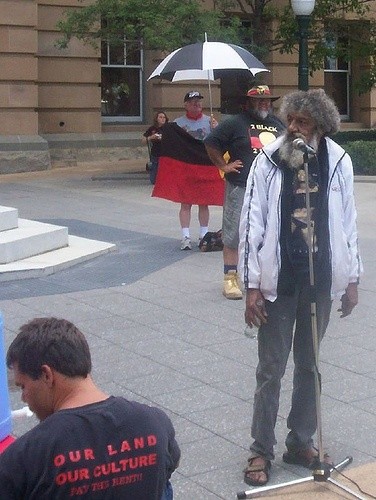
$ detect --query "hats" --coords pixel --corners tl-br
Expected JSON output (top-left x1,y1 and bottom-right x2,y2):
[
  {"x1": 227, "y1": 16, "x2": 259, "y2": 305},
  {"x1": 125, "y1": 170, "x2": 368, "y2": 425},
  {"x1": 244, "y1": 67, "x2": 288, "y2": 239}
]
[
  {"x1": 244, "y1": 81, "x2": 281, "y2": 101},
  {"x1": 184, "y1": 91, "x2": 204, "y2": 103}
]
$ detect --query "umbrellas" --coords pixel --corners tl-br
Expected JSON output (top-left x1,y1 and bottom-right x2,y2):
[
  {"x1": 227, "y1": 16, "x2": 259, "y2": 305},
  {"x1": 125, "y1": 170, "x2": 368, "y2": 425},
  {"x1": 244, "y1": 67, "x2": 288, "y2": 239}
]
[{"x1": 147, "y1": 32, "x2": 270, "y2": 128}]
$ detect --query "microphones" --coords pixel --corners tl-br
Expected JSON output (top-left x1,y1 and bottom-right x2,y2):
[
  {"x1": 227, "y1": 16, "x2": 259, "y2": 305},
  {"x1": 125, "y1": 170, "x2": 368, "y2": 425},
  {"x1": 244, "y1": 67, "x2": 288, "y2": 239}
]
[{"x1": 294, "y1": 138, "x2": 316, "y2": 156}]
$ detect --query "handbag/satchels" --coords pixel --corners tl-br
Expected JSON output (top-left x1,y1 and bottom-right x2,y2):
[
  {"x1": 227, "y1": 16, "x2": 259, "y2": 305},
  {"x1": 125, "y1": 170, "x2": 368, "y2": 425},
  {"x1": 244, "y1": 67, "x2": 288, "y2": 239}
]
[{"x1": 146, "y1": 162, "x2": 152, "y2": 173}]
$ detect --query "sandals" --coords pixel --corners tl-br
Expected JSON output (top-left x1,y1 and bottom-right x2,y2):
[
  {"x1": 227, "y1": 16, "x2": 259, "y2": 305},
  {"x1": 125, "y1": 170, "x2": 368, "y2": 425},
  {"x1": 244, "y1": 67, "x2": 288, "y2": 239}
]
[
  {"x1": 282, "y1": 445, "x2": 335, "y2": 471},
  {"x1": 242, "y1": 451, "x2": 271, "y2": 485}
]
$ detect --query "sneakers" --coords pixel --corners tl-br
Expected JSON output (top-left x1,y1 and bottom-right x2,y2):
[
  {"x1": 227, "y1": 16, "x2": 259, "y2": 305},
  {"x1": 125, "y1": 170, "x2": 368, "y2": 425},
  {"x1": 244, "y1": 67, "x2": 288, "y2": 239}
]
[
  {"x1": 180, "y1": 236, "x2": 192, "y2": 250},
  {"x1": 198, "y1": 238, "x2": 208, "y2": 249},
  {"x1": 222, "y1": 269, "x2": 243, "y2": 300}
]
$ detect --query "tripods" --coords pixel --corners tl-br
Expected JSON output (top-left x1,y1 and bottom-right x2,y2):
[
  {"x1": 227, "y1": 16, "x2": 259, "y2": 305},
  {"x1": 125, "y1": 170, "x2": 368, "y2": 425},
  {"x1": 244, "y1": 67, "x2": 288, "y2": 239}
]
[{"x1": 237, "y1": 153, "x2": 376, "y2": 500}]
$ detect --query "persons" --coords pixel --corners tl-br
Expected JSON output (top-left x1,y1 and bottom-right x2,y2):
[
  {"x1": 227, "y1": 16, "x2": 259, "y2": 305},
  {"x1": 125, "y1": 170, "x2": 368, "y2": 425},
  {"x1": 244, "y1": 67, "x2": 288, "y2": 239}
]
[
  {"x1": 142, "y1": 91, "x2": 218, "y2": 249},
  {"x1": 204, "y1": 83, "x2": 286, "y2": 300},
  {"x1": 238, "y1": 89, "x2": 364, "y2": 486},
  {"x1": 0, "y1": 314, "x2": 181, "y2": 500}
]
